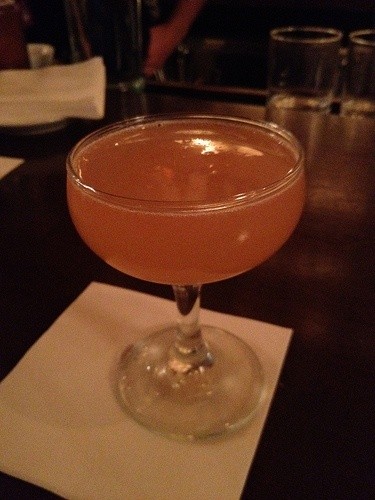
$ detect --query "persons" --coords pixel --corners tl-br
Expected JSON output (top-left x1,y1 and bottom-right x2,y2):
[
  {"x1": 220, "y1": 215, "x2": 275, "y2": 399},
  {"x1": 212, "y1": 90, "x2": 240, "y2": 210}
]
[{"x1": 20, "y1": 0, "x2": 206, "y2": 85}]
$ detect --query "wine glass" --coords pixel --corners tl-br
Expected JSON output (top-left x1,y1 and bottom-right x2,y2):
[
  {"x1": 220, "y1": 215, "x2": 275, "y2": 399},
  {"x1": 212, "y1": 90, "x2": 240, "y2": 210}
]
[{"x1": 65, "y1": 114, "x2": 305, "y2": 441}]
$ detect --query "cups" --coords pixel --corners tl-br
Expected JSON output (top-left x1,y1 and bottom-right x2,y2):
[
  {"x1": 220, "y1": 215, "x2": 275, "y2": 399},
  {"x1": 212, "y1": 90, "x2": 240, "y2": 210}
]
[
  {"x1": 266, "y1": 28, "x2": 342, "y2": 117},
  {"x1": 342, "y1": 31, "x2": 375, "y2": 119}
]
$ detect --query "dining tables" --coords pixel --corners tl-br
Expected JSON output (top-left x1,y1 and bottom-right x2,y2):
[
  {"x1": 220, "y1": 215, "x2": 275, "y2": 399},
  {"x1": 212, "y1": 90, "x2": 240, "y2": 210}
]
[{"x1": 1, "y1": 88, "x2": 375, "y2": 499}]
